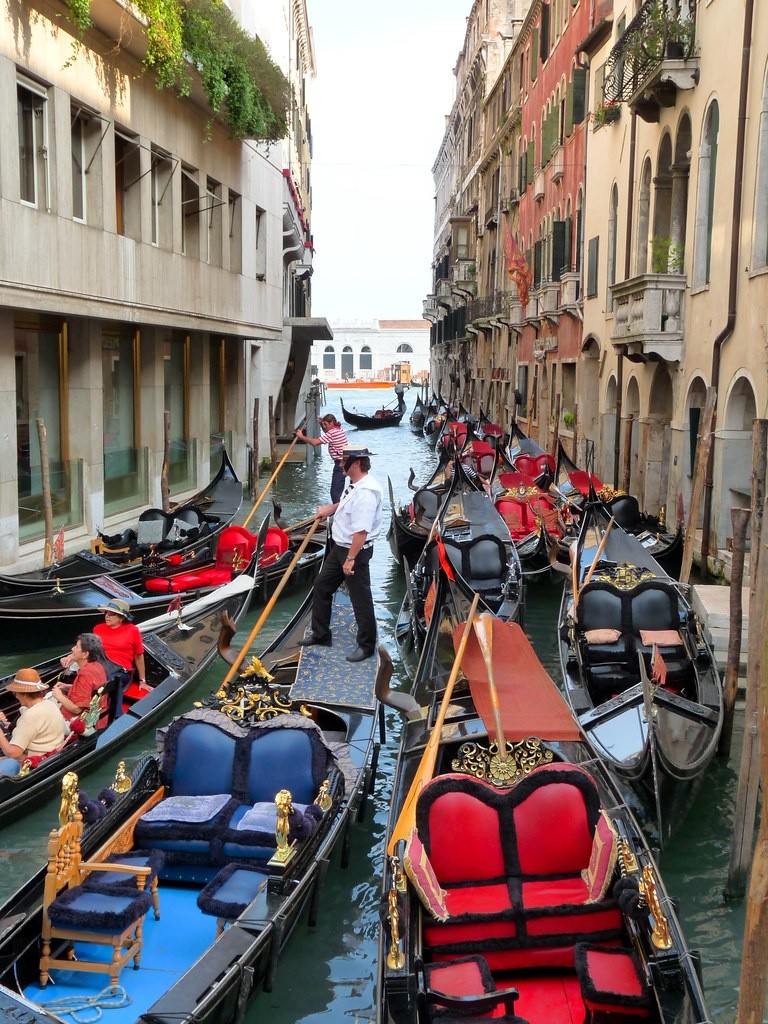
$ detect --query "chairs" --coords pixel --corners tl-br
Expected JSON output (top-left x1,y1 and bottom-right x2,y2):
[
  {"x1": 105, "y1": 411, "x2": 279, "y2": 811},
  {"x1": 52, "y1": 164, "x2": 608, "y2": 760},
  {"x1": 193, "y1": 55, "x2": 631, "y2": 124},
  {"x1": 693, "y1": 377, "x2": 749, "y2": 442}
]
[
  {"x1": 91, "y1": 530, "x2": 135, "y2": 563},
  {"x1": 141, "y1": 544, "x2": 203, "y2": 589},
  {"x1": 65, "y1": 672, "x2": 121, "y2": 743},
  {"x1": 417, "y1": 945, "x2": 524, "y2": 1024},
  {"x1": 195, "y1": 804, "x2": 324, "y2": 940},
  {"x1": 571, "y1": 921, "x2": 650, "y2": 1024},
  {"x1": 38, "y1": 810, "x2": 152, "y2": 991}
]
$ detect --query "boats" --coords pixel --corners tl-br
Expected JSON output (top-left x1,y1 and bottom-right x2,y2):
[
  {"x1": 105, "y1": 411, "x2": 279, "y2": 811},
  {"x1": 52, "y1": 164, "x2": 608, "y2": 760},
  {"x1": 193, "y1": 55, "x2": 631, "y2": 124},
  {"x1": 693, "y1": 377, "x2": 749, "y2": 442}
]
[
  {"x1": 17, "y1": 487, "x2": 69, "y2": 526},
  {"x1": 375, "y1": 518, "x2": 710, "y2": 1024},
  {"x1": 171, "y1": 431, "x2": 224, "y2": 456},
  {"x1": 1, "y1": 512, "x2": 381, "y2": 1024},
  {"x1": 0, "y1": 439, "x2": 244, "y2": 596},
  {"x1": 0, "y1": 498, "x2": 333, "y2": 638},
  {"x1": 556, "y1": 470, "x2": 724, "y2": 852},
  {"x1": 0, "y1": 512, "x2": 271, "y2": 829},
  {"x1": 325, "y1": 377, "x2": 397, "y2": 388},
  {"x1": 411, "y1": 379, "x2": 422, "y2": 387},
  {"x1": 386, "y1": 391, "x2": 684, "y2": 585},
  {"x1": 340, "y1": 397, "x2": 406, "y2": 428}
]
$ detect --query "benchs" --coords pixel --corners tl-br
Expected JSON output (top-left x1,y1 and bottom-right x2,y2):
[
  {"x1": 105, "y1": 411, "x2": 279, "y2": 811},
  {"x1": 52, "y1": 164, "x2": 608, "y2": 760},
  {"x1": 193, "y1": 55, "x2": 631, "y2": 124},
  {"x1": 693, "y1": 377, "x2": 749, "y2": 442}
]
[
  {"x1": 136, "y1": 502, "x2": 209, "y2": 552},
  {"x1": 420, "y1": 529, "x2": 508, "y2": 605},
  {"x1": 200, "y1": 524, "x2": 293, "y2": 585},
  {"x1": 515, "y1": 455, "x2": 556, "y2": 482},
  {"x1": 441, "y1": 432, "x2": 467, "y2": 448},
  {"x1": 590, "y1": 490, "x2": 645, "y2": 538},
  {"x1": 135, "y1": 707, "x2": 336, "y2": 887},
  {"x1": 462, "y1": 451, "x2": 495, "y2": 475},
  {"x1": 408, "y1": 758, "x2": 617, "y2": 961},
  {"x1": 576, "y1": 575, "x2": 690, "y2": 684},
  {"x1": 497, "y1": 491, "x2": 560, "y2": 547}
]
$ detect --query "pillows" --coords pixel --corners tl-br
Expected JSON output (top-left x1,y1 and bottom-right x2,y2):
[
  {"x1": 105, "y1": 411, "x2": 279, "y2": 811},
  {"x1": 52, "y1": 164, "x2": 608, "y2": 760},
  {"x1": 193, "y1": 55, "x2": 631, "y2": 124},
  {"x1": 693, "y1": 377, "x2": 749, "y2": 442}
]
[
  {"x1": 637, "y1": 629, "x2": 684, "y2": 649},
  {"x1": 165, "y1": 518, "x2": 196, "y2": 543},
  {"x1": 585, "y1": 628, "x2": 623, "y2": 646},
  {"x1": 578, "y1": 809, "x2": 614, "y2": 900},
  {"x1": 403, "y1": 826, "x2": 452, "y2": 924},
  {"x1": 136, "y1": 519, "x2": 163, "y2": 545}
]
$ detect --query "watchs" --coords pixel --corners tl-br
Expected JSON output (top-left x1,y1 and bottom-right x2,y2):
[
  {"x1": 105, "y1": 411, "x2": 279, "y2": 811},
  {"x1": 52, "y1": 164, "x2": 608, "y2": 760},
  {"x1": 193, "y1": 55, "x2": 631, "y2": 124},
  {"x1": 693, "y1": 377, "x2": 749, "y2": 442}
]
[
  {"x1": 346, "y1": 555, "x2": 355, "y2": 561},
  {"x1": 139, "y1": 679, "x2": 146, "y2": 682}
]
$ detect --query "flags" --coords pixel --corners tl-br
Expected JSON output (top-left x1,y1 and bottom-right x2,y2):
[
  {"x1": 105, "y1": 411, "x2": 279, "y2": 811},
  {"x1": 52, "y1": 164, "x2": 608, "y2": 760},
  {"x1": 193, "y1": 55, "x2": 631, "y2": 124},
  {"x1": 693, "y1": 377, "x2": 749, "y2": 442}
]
[
  {"x1": 650, "y1": 642, "x2": 667, "y2": 683},
  {"x1": 166, "y1": 595, "x2": 181, "y2": 613},
  {"x1": 501, "y1": 216, "x2": 534, "y2": 310},
  {"x1": 53, "y1": 526, "x2": 64, "y2": 564}
]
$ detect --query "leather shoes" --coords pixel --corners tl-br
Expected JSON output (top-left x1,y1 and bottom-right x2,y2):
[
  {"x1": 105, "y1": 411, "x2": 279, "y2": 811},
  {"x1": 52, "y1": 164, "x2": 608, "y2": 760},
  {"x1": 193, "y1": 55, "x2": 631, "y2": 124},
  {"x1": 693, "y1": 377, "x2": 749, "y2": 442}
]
[
  {"x1": 346, "y1": 646, "x2": 375, "y2": 662},
  {"x1": 297, "y1": 633, "x2": 333, "y2": 647}
]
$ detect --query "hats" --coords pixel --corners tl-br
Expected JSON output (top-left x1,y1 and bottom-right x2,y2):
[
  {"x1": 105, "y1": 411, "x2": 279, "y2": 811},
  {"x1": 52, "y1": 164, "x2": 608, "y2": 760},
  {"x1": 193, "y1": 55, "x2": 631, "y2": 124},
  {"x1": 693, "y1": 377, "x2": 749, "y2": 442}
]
[
  {"x1": 6, "y1": 669, "x2": 50, "y2": 694},
  {"x1": 333, "y1": 445, "x2": 377, "y2": 457},
  {"x1": 96, "y1": 599, "x2": 133, "y2": 621}
]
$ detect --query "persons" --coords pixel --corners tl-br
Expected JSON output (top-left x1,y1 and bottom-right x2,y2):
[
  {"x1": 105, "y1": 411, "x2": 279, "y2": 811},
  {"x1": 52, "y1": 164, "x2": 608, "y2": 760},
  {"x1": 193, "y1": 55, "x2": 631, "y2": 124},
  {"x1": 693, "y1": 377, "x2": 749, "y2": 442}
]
[
  {"x1": 344, "y1": 371, "x2": 349, "y2": 383},
  {"x1": 0, "y1": 669, "x2": 64, "y2": 778},
  {"x1": 296, "y1": 414, "x2": 349, "y2": 505},
  {"x1": 59, "y1": 599, "x2": 155, "y2": 719},
  {"x1": 34, "y1": 633, "x2": 109, "y2": 737},
  {"x1": 445, "y1": 453, "x2": 488, "y2": 491},
  {"x1": 298, "y1": 446, "x2": 383, "y2": 663},
  {"x1": 395, "y1": 380, "x2": 410, "y2": 412}
]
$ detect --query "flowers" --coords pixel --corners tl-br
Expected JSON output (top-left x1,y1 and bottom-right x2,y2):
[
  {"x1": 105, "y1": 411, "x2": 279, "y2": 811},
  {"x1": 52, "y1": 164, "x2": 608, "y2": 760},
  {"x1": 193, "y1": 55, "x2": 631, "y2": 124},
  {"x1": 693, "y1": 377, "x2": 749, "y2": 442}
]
[{"x1": 588, "y1": 98, "x2": 620, "y2": 128}]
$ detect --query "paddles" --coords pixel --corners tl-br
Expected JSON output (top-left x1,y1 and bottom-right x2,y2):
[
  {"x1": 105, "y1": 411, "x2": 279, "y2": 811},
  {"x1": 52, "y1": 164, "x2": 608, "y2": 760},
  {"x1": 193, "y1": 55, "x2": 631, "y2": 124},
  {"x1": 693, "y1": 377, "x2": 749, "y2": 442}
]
[
  {"x1": 282, "y1": 516, "x2": 317, "y2": 532},
  {"x1": 242, "y1": 405, "x2": 317, "y2": 528},
  {"x1": 548, "y1": 482, "x2": 584, "y2": 511},
  {"x1": 386, "y1": 591, "x2": 480, "y2": 858},
  {"x1": 193, "y1": 516, "x2": 322, "y2": 707},
  {"x1": 473, "y1": 612, "x2": 510, "y2": 763},
  {"x1": 382, "y1": 388, "x2": 410, "y2": 409},
  {"x1": 568, "y1": 540, "x2": 578, "y2": 621},
  {"x1": 560, "y1": 515, "x2": 615, "y2": 628}
]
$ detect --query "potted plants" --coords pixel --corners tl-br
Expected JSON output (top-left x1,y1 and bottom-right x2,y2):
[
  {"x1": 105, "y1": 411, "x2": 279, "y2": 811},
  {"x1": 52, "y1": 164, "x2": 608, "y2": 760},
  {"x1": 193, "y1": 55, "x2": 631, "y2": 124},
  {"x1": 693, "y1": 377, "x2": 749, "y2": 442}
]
[
  {"x1": 563, "y1": 412, "x2": 575, "y2": 431},
  {"x1": 615, "y1": 0, "x2": 702, "y2": 74}
]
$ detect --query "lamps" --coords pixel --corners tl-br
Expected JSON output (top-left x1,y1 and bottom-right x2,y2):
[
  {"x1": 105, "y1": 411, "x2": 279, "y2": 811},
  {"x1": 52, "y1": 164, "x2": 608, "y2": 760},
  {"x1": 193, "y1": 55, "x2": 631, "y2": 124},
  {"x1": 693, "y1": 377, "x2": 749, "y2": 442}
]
[{"x1": 290, "y1": 264, "x2": 314, "y2": 306}]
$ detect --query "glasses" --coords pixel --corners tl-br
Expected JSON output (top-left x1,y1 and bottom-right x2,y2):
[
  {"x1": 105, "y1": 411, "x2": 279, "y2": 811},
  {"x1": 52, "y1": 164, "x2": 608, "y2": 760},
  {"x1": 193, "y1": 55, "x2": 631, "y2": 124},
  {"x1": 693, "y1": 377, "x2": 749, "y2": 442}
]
[{"x1": 105, "y1": 610, "x2": 117, "y2": 617}]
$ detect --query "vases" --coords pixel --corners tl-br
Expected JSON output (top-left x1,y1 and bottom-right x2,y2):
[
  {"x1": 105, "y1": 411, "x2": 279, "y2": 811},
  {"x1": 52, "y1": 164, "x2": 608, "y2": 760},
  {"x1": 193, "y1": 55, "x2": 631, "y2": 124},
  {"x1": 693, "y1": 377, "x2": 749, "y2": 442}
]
[{"x1": 608, "y1": 108, "x2": 618, "y2": 120}]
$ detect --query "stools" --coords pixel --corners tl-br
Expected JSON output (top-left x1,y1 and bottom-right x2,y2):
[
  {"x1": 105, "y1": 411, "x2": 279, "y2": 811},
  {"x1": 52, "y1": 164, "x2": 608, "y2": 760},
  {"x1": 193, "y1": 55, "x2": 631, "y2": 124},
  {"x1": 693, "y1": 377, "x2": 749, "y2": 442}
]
[{"x1": 84, "y1": 847, "x2": 165, "y2": 922}]
